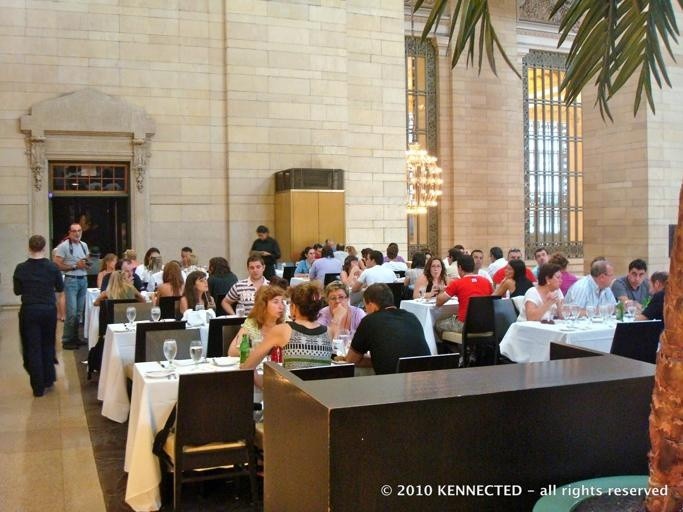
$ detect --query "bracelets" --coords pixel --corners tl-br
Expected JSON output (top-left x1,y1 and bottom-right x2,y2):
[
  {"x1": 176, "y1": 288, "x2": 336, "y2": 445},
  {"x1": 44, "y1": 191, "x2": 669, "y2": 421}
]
[{"x1": 72, "y1": 265, "x2": 78, "y2": 270}]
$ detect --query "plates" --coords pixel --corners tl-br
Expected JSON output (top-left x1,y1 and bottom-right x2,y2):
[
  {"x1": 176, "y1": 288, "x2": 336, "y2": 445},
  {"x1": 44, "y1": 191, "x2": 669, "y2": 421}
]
[
  {"x1": 211, "y1": 357, "x2": 237, "y2": 367},
  {"x1": 144, "y1": 370, "x2": 176, "y2": 377}
]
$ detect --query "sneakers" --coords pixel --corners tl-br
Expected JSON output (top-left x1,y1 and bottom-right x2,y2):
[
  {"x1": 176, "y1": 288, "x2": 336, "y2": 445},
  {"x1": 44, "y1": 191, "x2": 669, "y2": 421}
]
[
  {"x1": 44, "y1": 379, "x2": 54, "y2": 388},
  {"x1": 63, "y1": 342, "x2": 78, "y2": 349},
  {"x1": 32, "y1": 383, "x2": 45, "y2": 397},
  {"x1": 73, "y1": 337, "x2": 87, "y2": 346}
]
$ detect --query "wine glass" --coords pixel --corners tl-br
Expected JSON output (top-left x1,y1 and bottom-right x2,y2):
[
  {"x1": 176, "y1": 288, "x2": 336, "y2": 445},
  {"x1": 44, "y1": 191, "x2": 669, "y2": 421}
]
[
  {"x1": 195, "y1": 303, "x2": 205, "y2": 312},
  {"x1": 414, "y1": 285, "x2": 458, "y2": 304},
  {"x1": 545, "y1": 295, "x2": 652, "y2": 330},
  {"x1": 207, "y1": 302, "x2": 217, "y2": 313},
  {"x1": 189, "y1": 339, "x2": 202, "y2": 372},
  {"x1": 126, "y1": 306, "x2": 137, "y2": 327},
  {"x1": 330, "y1": 329, "x2": 358, "y2": 359},
  {"x1": 162, "y1": 339, "x2": 177, "y2": 369},
  {"x1": 150, "y1": 306, "x2": 161, "y2": 322}
]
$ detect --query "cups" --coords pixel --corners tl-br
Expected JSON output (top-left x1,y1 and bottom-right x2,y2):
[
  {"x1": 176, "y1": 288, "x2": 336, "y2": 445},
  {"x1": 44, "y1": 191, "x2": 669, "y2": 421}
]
[
  {"x1": 281, "y1": 262, "x2": 286, "y2": 271},
  {"x1": 236, "y1": 304, "x2": 246, "y2": 318}
]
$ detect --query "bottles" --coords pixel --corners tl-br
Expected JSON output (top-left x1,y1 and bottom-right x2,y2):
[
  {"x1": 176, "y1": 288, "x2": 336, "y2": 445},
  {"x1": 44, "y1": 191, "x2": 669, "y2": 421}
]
[
  {"x1": 270, "y1": 345, "x2": 283, "y2": 363},
  {"x1": 240, "y1": 334, "x2": 250, "y2": 365}
]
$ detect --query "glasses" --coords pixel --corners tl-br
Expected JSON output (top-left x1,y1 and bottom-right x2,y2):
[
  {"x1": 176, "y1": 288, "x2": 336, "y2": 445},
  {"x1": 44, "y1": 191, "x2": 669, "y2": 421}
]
[{"x1": 328, "y1": 295, "x2": 349, "y2": 300}]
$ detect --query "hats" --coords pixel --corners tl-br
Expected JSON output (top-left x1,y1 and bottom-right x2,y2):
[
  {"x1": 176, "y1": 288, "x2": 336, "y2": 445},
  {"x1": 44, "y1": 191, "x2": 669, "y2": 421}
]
[{"x1": 89, "y1": 246, "x2": 102, "y2": 256}]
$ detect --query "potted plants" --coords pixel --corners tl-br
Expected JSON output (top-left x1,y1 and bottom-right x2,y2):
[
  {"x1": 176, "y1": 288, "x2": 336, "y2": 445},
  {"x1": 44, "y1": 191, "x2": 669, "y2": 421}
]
[{"x1": 417, "y1": 0, "x2": 683, "y2": 512}]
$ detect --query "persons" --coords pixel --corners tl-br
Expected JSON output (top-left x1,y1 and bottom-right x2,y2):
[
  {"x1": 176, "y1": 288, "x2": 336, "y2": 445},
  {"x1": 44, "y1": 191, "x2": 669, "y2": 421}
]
[
  {"x1": 53, "y1": 222, "x2": 94, "y2": 350},
  {"x1": 247, "y1": 225, "x2": 281, "y2": 281},
  {"x1": 51, "y1": 235, "x2": 65, "y2": 322},
  {"x1": 84, "y1": 239, "x2": 671, "y2": 424},
  {"x1": 12, "y1": 234, "x2": 65, "y2": 397}
]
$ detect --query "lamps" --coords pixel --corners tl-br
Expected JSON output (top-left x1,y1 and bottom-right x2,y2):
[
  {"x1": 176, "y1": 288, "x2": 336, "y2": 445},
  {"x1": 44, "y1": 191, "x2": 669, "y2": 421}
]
[{"x1": 405, "y1": 0, "x2": 444, "y2": 216}]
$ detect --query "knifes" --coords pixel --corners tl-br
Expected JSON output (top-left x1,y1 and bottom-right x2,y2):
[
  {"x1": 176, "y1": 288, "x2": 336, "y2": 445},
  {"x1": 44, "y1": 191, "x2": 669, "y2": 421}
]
[
  {"x1": 123, "y1": 322, "x2": 127, "y2": 327},
  {"x1": 157, "y1": 360, "x2": 165, "y2": 367}
]
